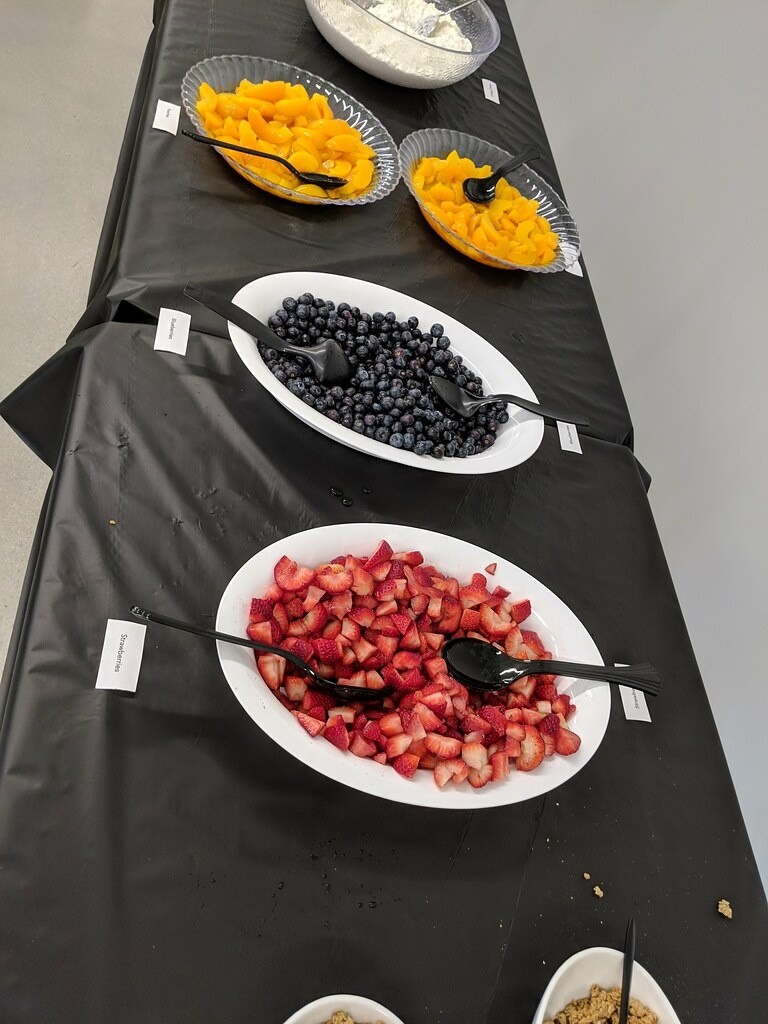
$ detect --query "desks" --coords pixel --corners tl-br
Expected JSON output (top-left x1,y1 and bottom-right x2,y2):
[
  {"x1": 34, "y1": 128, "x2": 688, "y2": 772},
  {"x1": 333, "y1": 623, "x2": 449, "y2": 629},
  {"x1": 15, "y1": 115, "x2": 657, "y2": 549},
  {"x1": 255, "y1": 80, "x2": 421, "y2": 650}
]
[
  {"x1": 68, "y1": 0, "x2": 633, "y2": 452},
  {"x1": 1, "y1": 320, "x2": 768, "y2": 1024}
]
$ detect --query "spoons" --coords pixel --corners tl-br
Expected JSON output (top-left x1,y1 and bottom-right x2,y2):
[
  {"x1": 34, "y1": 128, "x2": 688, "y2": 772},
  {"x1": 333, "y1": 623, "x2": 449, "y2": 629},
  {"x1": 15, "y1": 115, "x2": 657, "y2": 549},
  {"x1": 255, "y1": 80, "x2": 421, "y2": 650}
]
[
  {"x1": 428, "y1": 375, "x2": 590, "y2": 427},
  {"x1": 182, "y1": 279, "x2": 357, "y2": 388},
  {"x1": 181, "y1": 129, "x2": 348, "y2": 188},
  {"x1": 125, "y1": 605, "x2": 397, "y2": 699},
  {"x1": 416, "y1": 0, "x2": 478, "y2": 35},
  {"x1": 463, "y1": 144, "x2": 540, "y2": 202},
  {"x1": 442, "y1": 636, "x2": 662, "y2": 696}
]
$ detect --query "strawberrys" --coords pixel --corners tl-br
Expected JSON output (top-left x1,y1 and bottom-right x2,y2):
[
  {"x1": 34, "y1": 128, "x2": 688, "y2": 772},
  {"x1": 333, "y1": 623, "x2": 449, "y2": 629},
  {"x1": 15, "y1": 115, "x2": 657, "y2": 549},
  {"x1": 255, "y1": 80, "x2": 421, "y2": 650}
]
[{"x1": 246, "y1": 539, "x2": 582, "y2": 788}]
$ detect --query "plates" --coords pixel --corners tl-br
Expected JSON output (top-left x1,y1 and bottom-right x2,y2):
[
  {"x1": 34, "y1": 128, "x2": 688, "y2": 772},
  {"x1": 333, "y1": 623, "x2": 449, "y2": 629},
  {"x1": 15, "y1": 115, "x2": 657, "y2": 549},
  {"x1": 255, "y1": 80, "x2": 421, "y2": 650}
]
[
  {"x1": 215, "y1": 523, "x2": 611, "y2": 809},
  {"x1": 528, "y1": 946, "x2": 683, "y2": 1024},
  {"x1": 396, "y1": 128, "x2": 581, "y2": 274},
  {"x1": 180, "y1": 54, "x2": 402, "y2": 206},
  {"x1": 277, "y1": 994, "x2": 404, "y2": 1024},
  {"x1": 227, "y1": 271, "x2": 545, "y2": 474}
]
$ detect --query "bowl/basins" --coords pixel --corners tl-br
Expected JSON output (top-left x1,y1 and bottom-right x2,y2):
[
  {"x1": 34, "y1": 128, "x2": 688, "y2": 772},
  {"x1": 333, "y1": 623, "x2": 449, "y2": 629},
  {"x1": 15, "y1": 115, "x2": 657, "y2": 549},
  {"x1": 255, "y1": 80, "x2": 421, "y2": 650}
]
[{"x1": 304, "y1": 0, "x2": 502, "y2": 88}]
